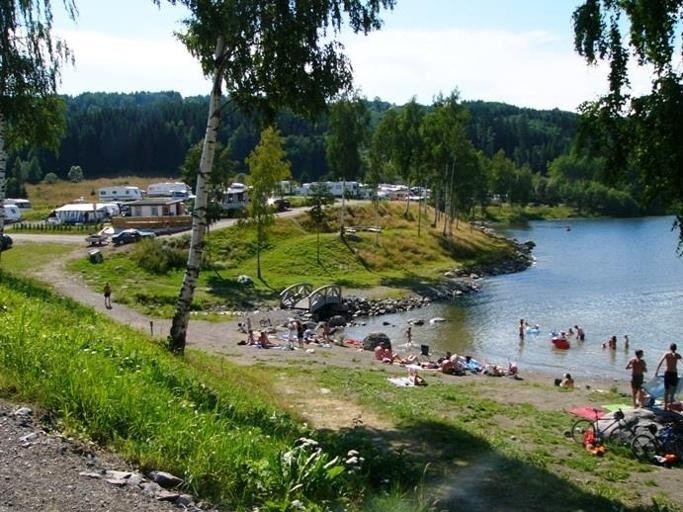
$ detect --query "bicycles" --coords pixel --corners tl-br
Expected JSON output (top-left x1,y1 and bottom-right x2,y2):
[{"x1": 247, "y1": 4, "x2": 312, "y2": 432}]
[{"x1": 572, "y1": 408, "x2": 683, "y2": 464}]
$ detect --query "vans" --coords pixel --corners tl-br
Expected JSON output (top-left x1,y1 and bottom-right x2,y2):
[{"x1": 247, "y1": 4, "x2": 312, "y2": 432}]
[{"x1": 3, "y1": 198, "x2": 32, "y2": 221}]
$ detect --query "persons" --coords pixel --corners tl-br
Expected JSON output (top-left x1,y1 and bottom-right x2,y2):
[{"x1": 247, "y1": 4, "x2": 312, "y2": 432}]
[
  {"x1": 103, "y1": 282, "x2": 112, "y2": 309},
  {"x1": 374, "y1": 326, "x2": 506, "y2": 386},
  {"x1": 553, "y1": 324, "x2": 629, "y2": 350},
  {"x1": 519, "y1": 319, "x2": 525, "y2": 339},
  {"x1": 655, "y1": 344, "x2": 682, "y2": 411},
  {"x1": 239, "y1": 317, "x2": 304, "y2": 351},
  {"x1": 321, "y1": 323, "x2": 330, "y2": 340},
  {"x1": 560, "y1": 372, "x2": 574, "y2": 387},
  {"x1": 626, "y1": 350, "x2": 648, "y2": 408}
]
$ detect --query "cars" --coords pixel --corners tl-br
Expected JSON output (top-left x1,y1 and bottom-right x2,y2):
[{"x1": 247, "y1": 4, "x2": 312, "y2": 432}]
[
  {"x1": 268, "y1": 198, "x2": 290, "y2": 212},
  {"x1": 111, "y1": 228, "x2": 156, "y2": 245}
]
